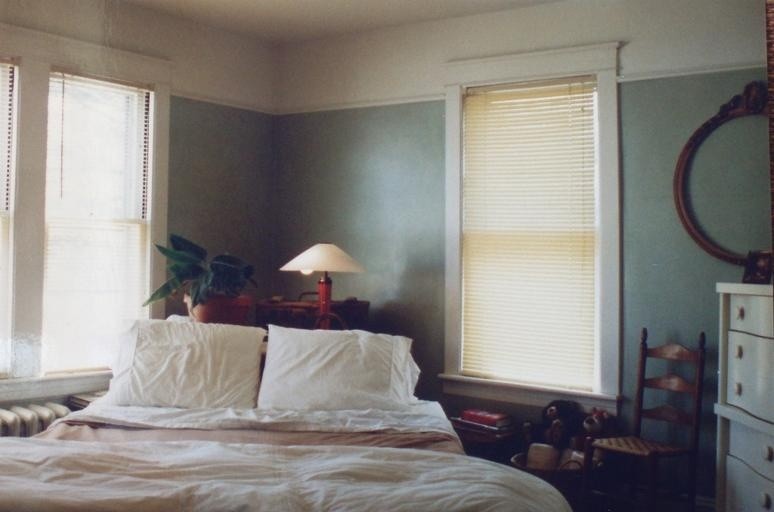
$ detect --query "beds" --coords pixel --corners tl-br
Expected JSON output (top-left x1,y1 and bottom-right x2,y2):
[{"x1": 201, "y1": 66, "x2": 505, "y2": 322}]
[{"x1": 0, "y1": 399, "x2": 573, "y2": 512}]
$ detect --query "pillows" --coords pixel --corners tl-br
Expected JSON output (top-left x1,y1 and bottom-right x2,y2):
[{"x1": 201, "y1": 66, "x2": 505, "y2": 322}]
[
  {"x1": 105, "y1": 314, "x2": 267, "y2": 409},
  {"x1": 257, "y1": 323, "x2": 420, "y2": 411}
]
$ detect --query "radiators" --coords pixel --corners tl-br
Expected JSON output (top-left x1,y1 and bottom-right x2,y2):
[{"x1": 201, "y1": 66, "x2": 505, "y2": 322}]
[{"x1": 1, "y1": 402, "x2": 71, "y2": 437}]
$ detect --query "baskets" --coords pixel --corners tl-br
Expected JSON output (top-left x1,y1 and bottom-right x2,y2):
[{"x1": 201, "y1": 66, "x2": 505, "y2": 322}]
[{"x1": 509, "y1": 446, "x2": 590, "y2": 512}]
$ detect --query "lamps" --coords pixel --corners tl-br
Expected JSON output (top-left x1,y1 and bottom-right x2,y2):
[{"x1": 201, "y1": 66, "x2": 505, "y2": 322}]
[{"x1": 278, "y1": 243, "x2": 365, "y2": 329}]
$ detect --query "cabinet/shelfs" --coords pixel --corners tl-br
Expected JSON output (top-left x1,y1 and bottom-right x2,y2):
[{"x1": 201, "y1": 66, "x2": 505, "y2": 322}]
[{"x1": 712, "y1": 284, "x2": 774, "y2": 512}]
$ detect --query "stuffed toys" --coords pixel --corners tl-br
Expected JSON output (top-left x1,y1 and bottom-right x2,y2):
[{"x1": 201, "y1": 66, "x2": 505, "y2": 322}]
[{"x1": 522, "y1": 399, "x2": 609, "y2": 459}]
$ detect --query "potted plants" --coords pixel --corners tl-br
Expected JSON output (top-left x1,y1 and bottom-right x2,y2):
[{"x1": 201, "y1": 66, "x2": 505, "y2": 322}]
[{"x1": 139, "y1": 232, "x2": 258, "y2": 325}]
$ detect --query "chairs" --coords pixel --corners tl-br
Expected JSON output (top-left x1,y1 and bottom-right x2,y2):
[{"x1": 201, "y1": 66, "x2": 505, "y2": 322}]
[{"x1": 592, "y1": 327, "x2": 706, "y2": 512}]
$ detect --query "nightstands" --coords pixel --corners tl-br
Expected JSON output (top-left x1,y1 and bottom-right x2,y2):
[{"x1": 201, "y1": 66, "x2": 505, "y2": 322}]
[
  {"x1": 69, "y1": 388, "x2": 108, "y2": 408},
  {"x1": 450, "y1": 416, "x2": 534, "y2": 449}
]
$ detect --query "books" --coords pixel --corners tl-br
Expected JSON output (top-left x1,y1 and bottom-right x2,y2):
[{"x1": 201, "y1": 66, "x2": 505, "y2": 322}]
[{"x1": 450, "y1": 408, "x2": 516, "y2": 435}]
